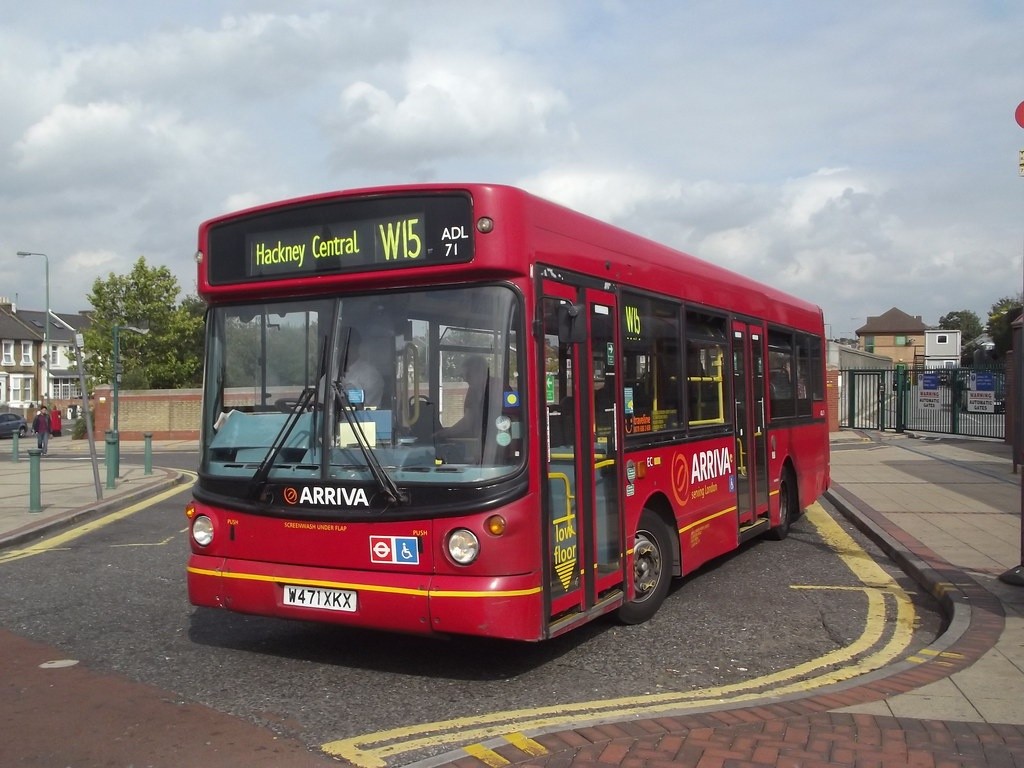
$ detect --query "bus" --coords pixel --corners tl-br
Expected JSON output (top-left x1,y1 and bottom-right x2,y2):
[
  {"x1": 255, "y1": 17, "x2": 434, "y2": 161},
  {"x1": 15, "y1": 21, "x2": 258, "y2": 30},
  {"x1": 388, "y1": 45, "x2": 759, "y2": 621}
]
[{"x1": 184, "y1": 182, "x2": 833, "y2": 647}]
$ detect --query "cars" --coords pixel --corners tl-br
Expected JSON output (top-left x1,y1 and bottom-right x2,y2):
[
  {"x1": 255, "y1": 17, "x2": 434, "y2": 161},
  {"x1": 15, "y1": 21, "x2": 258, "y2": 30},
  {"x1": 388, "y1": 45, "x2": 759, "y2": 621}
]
[{"x1": 0, "y1": 412, "x2": 28, "y2": 439}]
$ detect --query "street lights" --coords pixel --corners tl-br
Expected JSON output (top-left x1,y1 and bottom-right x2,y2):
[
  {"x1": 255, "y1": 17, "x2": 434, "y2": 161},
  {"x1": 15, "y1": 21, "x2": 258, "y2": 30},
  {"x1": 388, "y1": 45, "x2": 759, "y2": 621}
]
[{"x1": 17, "y1": 251, "x2": 50, "y2": 417}]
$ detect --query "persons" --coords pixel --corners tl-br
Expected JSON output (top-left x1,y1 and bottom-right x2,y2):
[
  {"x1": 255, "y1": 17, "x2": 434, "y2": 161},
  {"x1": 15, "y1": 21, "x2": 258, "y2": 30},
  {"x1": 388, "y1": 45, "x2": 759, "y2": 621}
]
[
  {"x1": 50, "y1": 404, "x2": 62, "y2": 437},
  {"x1": 438, "y1": 354, "x2": 519, "y2": 467},
  {"x1": 33, "y1": 405, "x2": 52, "y2": 456},
  {"x1": 309, "y1": 325, "x2": 384, "y2": 411},
  {"x1": 548, "y1": 351, "x2": 807, "y2": 444}
]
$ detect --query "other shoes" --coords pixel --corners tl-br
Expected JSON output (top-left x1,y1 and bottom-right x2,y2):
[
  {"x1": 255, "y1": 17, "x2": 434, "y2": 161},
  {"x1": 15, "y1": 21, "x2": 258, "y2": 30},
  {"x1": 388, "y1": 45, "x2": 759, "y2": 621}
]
[{"x1": 41, "y1": 452, "x2": 46, "y2": 456}]
